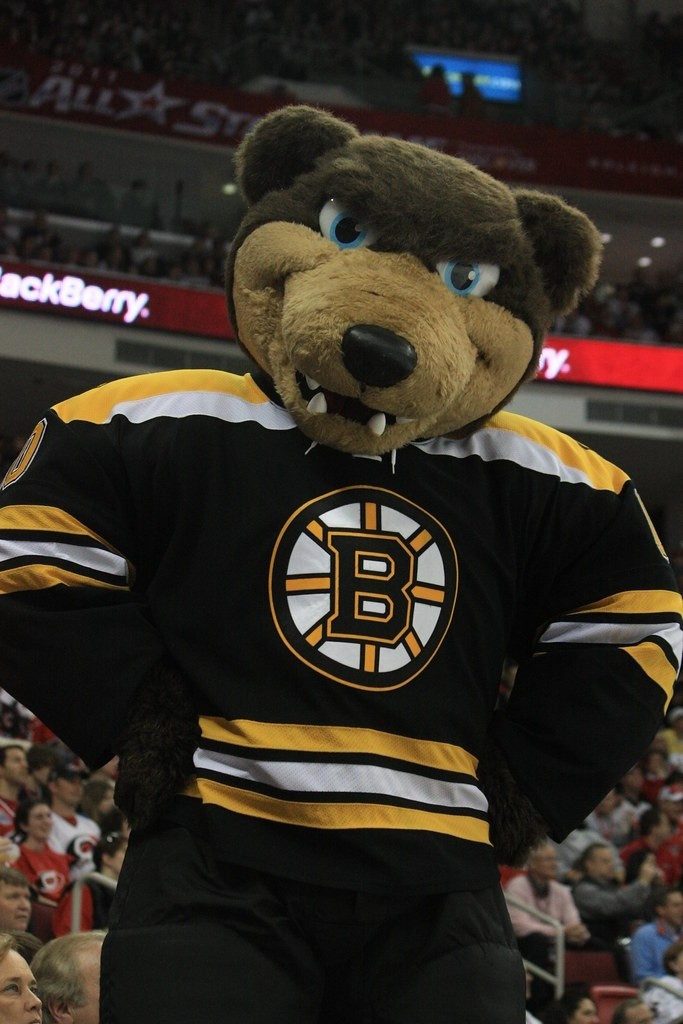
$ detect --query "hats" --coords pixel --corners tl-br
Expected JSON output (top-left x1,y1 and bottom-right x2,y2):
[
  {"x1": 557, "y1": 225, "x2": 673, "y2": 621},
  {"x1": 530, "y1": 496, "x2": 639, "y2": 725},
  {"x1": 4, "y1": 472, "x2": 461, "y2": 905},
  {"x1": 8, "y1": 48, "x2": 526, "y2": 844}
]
[{"x1": 48, "y1": 748, "x2": 90, "y2": 782}]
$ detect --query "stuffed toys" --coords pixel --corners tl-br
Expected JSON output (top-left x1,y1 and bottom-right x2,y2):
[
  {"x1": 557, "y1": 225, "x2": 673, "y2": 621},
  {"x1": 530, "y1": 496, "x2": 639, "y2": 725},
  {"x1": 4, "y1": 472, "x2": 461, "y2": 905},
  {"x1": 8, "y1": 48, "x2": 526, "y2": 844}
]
[{"x1": 0, "y1": 103, "x2": 683, "y2": 1024}]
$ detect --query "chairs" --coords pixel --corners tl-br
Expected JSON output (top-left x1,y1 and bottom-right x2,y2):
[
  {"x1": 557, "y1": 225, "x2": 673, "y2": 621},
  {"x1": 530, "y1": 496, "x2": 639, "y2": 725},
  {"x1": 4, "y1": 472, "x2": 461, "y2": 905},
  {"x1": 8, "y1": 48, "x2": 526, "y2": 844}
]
[
  {"x1": 26, "y1": 899, "x2": 56, "y2": 943},
  {"x1": 565, "y1": 950, "x2": 621, "y2": 994},
  {"x1": 591, "y1": 986, "x2": 638, "y2": 1024}
]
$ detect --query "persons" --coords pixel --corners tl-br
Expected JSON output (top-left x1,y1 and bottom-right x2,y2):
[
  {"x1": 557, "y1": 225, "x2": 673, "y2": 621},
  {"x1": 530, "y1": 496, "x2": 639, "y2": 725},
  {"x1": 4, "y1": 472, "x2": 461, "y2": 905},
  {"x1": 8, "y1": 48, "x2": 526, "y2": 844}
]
[
  {"x1": 0, "y1": 689, "x2": 131, "y2": 1024},
  {"x1": 496, "y1": 698, "x2": 683, "y2": 1024},
  {"x1": 0, "y1": 0, "x2": 683, "y2": 345}
]
